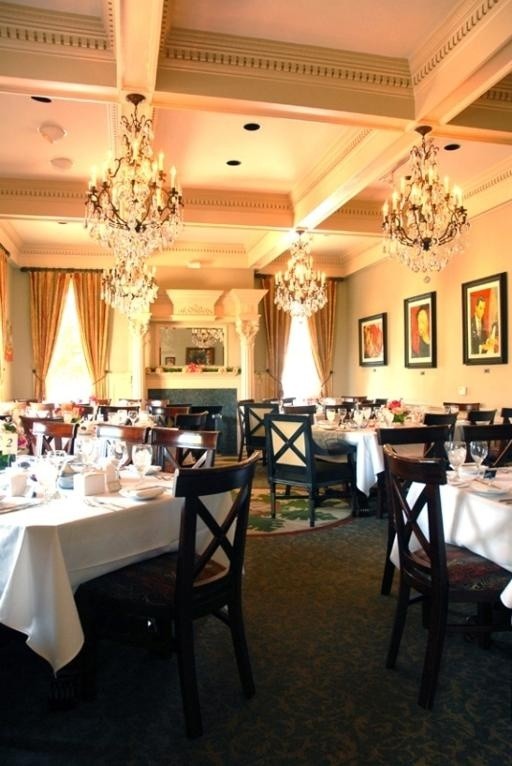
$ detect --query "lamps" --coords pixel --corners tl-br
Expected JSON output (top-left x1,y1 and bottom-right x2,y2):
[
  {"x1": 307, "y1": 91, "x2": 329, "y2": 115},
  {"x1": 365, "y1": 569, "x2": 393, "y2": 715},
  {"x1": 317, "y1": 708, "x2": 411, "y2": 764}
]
[
  {"x1": 83, "y1": 93, "x2": 184, "y2": 266},
  {"x1": 380, "y1": 126, "x2": 470, "y2": 284},
  {"x1": 273, "y1": 227, "x2": 328, "y2": 324},
  {"x1": 101, "y1": 262, "x2": 159, "y2": 337},
  {"x1": 191, "y1": 329, "x2": 223, "y2": 349}
]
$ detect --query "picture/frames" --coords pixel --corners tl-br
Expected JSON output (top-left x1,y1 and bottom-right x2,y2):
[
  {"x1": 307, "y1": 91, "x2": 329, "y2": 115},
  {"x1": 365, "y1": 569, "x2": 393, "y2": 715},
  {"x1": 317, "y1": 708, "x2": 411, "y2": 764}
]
[
  {"x1": 185, "y1": 347, "x2": 215, "y2": 365},
  {"x1": 358, "y1": 312, "x2": 387, "y2": 367},
  {"x1": 461, "y1": 271, "x2": 508, "y2": 365},
  {"x1": 165, "y1": 357, "x2": 175, "y2": 365},
  {"x1": 404, "y1": 291, "x2": 437, "y2": 369}
]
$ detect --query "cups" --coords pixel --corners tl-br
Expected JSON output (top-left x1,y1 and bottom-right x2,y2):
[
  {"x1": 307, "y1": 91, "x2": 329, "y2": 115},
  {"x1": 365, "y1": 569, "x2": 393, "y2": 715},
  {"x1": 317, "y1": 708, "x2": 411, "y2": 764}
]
[{"x1": 15, "y1": 435, "x2": 151, "y2": 502}]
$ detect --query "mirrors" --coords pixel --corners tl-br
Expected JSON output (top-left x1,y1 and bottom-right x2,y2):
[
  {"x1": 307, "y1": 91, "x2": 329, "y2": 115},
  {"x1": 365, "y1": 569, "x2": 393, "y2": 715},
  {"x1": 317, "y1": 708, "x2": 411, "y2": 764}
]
[{"x1": 155, "y1": 324, "x2": 227, "y2": 369}]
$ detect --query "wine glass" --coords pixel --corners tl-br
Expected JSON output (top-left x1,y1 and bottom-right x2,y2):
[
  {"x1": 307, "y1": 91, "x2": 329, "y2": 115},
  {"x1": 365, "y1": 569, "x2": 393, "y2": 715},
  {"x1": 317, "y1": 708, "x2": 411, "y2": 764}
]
[
  {"x1": 326, "y1": 406, "x2": 394, "y2": 430},
  {"x1": 447, "y1": 441, "x2": 466, "y2": 482},
  {"x1": 61, "y1": 409, "x2": 141, "y2": 426},
  {"x1": 470, "y1": 440, "x2": 488, "y2": 479}
]
